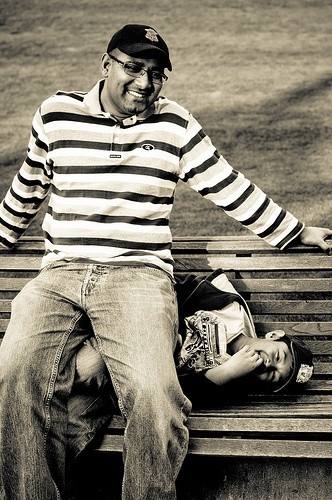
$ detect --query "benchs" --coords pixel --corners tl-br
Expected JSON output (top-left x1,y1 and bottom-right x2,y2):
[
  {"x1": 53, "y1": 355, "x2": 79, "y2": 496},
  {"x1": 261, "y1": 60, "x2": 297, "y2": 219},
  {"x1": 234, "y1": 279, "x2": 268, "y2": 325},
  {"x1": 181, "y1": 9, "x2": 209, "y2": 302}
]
[{"x1": 0, "y1": 231, "x2": 332, "y2": 460}]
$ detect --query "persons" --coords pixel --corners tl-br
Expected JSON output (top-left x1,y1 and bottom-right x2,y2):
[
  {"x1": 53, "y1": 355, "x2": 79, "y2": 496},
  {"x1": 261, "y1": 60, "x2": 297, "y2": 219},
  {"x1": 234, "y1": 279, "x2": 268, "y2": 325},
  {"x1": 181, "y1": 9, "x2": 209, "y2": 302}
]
[
  {"x1": 1, "y1": 24, "x2": 332, "y2": 500},
  {"x1": 64, "y1": 268, "x2": 314, "y2": 458}
]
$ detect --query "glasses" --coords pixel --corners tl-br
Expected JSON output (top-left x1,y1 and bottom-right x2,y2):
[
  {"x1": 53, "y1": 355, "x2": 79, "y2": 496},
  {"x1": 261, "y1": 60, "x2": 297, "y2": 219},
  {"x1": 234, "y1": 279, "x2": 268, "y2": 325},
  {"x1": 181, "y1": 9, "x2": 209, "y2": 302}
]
[{"x1": 108, "y1": 54, "x2": 168, "y2": 85}]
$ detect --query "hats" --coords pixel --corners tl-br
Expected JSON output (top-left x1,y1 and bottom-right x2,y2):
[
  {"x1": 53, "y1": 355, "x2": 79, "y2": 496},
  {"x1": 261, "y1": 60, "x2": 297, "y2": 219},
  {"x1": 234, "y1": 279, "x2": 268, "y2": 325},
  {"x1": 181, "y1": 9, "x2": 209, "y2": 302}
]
[
  {"x1": 274, "y1": 337, "x2": 313, "y2": 393},
  {"x1": 107, "y1": 23, "x2": 173, "y2": 72}
]
